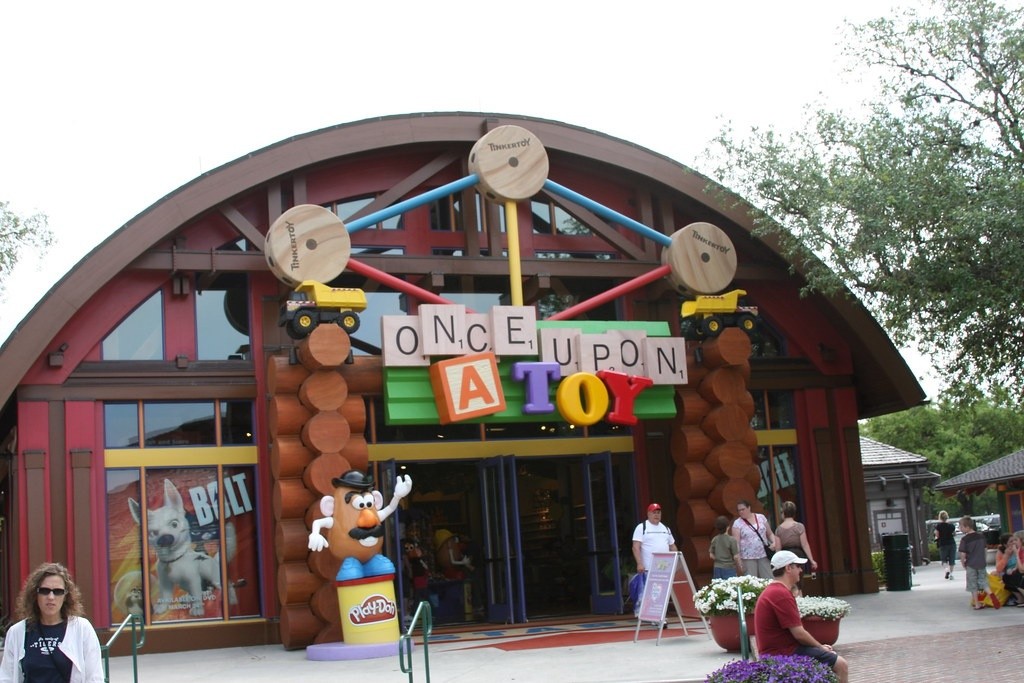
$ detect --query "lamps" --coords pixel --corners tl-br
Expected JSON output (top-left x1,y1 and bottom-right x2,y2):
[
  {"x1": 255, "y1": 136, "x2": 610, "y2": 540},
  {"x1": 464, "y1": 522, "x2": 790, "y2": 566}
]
[
  {"x1": 818, "y1": 344, "x2": 837, "y2": 361},
  {"x1": 172, "y1": 274, "x2": 191, "y2": 296},
  {"x1": 48, "y1": 343, "x2": 67, "y2": 366}
]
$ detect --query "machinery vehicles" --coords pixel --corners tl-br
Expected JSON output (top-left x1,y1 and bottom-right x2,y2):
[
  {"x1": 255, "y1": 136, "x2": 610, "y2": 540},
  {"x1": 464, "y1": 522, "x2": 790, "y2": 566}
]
[
  {"x1": 680, "y1": 289, "x2": 759, "y2": 341},
  {"x1": 278, "y1": 280, "x2": 367, "y2": 339}
]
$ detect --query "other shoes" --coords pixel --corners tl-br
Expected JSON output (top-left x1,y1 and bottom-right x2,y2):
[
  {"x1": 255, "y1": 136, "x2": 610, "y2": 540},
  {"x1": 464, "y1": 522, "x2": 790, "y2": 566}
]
[
  {"x1": 653, "y1": 620, "x2": 669, "y2": 628},
  {"x1": 945, "y1": 571, "x2": 950, "y2": 579},
  {"x1": 950, "y1": 576, "x2": 954, "y2": 580},
  {"x1": 1013, "y1": 601, "x2": 1024, "y2": 607},
  {"x1": 1007, "y1": 597, "x2": 1016, "y2": 606},
  {"x1": 990, "y1": 593, "x2": 1000, "y2": 609},
  {"x1": 975, "y1": 603, "x2": 983, "y2": 610}
]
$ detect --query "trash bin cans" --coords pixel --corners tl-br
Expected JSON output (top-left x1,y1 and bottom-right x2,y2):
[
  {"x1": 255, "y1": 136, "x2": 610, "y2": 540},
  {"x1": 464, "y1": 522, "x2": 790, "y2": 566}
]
[{"x1": 880, "y1": 534, "x2": 912, "y2": 591}]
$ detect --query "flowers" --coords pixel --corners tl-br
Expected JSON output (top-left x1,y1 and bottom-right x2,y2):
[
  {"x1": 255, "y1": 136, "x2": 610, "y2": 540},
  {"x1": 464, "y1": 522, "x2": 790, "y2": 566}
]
[
  {"x1": 692, "y1": 575, "x2": 774, "y2": 615},
  {"x1": 706, "y1": 654, "x2": 838, "y2": 683},
  {"x1": 793, "y1": 595, "x2": 850, "y2": 621}
]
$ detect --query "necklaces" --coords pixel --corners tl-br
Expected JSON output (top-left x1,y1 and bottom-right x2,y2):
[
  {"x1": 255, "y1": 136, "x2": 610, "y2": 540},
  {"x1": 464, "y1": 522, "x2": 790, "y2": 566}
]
[{"x1": 743, "y1": 514, "x2": 759, "y2": 532}]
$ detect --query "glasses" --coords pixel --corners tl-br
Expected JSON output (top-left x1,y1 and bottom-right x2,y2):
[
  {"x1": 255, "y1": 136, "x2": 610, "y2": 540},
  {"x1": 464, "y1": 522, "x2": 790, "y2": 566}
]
[{"x1": 36, "y1": 587, "x2": 68, "y2": 595}]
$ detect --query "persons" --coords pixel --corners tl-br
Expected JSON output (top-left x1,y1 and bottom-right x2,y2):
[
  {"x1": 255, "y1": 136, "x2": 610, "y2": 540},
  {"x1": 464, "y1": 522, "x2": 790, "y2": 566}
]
[
  {"x1": 709, "y1": 517, "x2": 740, "y2": 577},
  {"x1": 934, "y1": 510, "x2": 956, "y2": 580},
  {"x1": 632, "y1": 504, "x2": 679, "y2": 628},
  {"x1": 774, "y1": 501, "x2": 817, "y2": 596},
  {"x1": 995, "y1": 530, "x2": 1024, "y2": 607},
  {"x1": 958, "y1": 516, "x2": 1000, "y2": 609},
  {"x1": 755, "y1": 550, "x2": 849, "y2": 683},
  {"x1": 0, "y1": 562, "x2": 104, "y2": 683},
  {"x1": 732, "y1": 499, "x2": 775, "y2": 579}
]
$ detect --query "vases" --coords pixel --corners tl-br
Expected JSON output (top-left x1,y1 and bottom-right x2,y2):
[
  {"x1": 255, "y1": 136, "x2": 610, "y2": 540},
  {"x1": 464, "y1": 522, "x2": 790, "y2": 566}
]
[
  {"x1": 800, "y1": 615, "x2": 843, "y2": 645},
  {"x1": 708, "y1": 613, "x2": 754, "y2": 652}
]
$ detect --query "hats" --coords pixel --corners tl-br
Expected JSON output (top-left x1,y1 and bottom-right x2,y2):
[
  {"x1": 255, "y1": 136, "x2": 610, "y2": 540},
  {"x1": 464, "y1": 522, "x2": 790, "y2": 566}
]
[
  {"x1": 648, "y1": 504, "x2": 662, "y2": 512},
  {"x1": 770, "y1": 550, "x2": 808, "y2": 572}
]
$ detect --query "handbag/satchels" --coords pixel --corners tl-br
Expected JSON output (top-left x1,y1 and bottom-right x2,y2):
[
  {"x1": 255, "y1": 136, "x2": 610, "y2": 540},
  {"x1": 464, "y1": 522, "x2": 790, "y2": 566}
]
[
  {"x1": 977, "y1": 573, "x2": 1010, "y2": 607},
  {"x1": 629, "y1": 572, "x2": 648, "y2": 618},
  {"x1": 764, "y1": 544, "x2": 775, "y2": 560}
]
